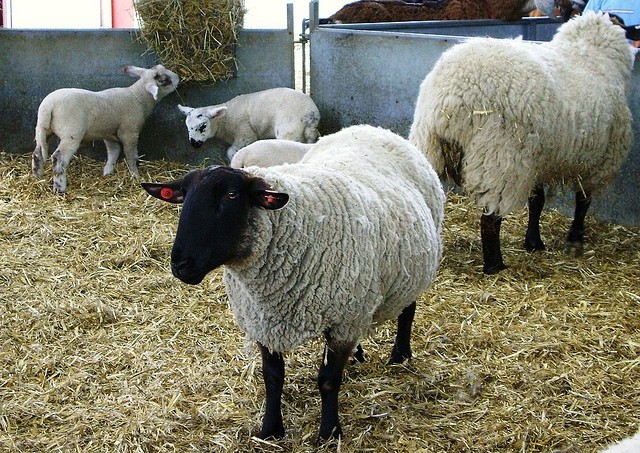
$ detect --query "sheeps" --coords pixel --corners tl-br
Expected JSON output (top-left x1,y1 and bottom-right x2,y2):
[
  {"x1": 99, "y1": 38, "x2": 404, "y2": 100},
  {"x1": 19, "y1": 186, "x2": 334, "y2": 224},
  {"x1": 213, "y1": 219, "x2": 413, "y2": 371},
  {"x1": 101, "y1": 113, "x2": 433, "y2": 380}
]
[
  {"x1": 226, "y1": 136, "x2": 316, "y2": 170},
  {"x1": 407, "y1": 9, "x2": 635, "y2": 275},
  {"x1": 32, "y1": 62, "x2": 179, "y2": 196},
  {"x1": 177, "y1": 85, "x2": 323, "y2": 162},
  {"x1": 137, "y1": 124, "x2": 447, "y2": 441}
]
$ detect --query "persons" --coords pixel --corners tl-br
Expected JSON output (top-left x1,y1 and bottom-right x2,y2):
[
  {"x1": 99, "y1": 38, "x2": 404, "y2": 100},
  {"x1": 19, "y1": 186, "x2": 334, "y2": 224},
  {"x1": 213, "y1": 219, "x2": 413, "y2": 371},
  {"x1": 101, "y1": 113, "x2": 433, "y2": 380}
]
[{"x1": 582, "y1": 0, "x2": 640, "y2": 47}]
